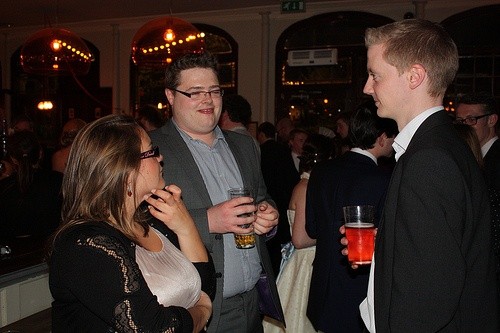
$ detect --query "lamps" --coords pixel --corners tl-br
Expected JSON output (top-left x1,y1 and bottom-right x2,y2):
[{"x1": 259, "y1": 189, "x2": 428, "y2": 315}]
[{"x1": 19, "y1": 0, "x2": 208, "y2": 76}]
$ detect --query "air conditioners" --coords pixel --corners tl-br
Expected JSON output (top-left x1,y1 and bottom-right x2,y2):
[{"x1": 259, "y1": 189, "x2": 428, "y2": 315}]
[{"x1": 288, "y1": 47, "x2": 339, "y2": 67}]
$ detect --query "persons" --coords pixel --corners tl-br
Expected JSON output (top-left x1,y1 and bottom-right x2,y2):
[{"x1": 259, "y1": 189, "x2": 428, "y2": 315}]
[
  {"x1": 0, "y1": 19, "x2": 500, "y2": 333},
  {"x1": 306, "y1": 110, "x2": 399, "y2": 333},
  {"x1": 456, "y1": 93, "x2": 500, "y2": 234},
  {"x1": 361, "y1": 19, "x2": 496, "y2": 333},
  {"x1": 146, "y1": 52, "x2": 286, "y2": 333},
  {"x1": 261, "y1": 134, "x2": 337, "y2": 333},
  {"x1": 49, "y1": 115, "x2": 216, "y2": 333}
]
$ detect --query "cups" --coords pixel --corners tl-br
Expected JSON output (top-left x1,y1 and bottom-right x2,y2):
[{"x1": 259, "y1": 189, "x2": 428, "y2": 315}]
[
  {"x1": 343, "y1": 205, "x2": 375, "y2": 264},
  {"x1": 228, "y1": 188, "x2": 256, "y2": 248}
]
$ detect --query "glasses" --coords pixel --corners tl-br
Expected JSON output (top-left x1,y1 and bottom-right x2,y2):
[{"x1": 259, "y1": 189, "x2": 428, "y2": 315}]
[
  {"x1": 140, "y1": 144, "x2": 160, "y2": 159},
  {"x1": 170, "y1": 87, "x2": 224, "y2": 100},
  {"x1": 455, "y1": 112, "x2": 495, "y2": 126}
]
